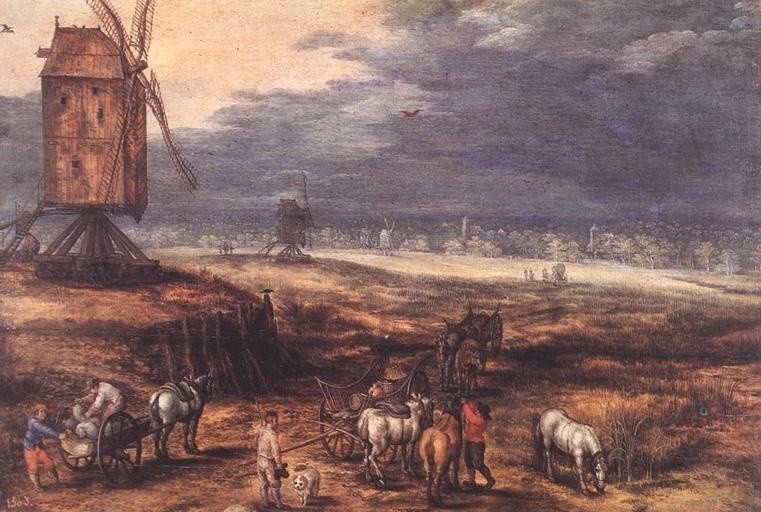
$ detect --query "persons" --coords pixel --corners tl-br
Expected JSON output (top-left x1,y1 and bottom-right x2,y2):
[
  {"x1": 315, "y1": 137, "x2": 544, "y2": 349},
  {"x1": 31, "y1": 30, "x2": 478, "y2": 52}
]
[
  {"x1": 522, "y1": 261, "x2": 569, "y2": 283},
  {"x1": 74, "y1": 375, "x2": 128, "y2": 437},
  {"x1": 22, "y1": 403, "x2": 66, "y2": 493},
  {"x1": 460, "y1": 395, "x2": 496, "y2": 488},
  {"x1": 252, "y1": 410, "x2": 291, "y2": 510}
]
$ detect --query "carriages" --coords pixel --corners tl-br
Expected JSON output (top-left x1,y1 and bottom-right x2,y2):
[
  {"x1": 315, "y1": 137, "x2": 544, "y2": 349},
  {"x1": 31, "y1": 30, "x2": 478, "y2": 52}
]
[
  {"x1": 51, "y1": 372, "x2": 214, "y2": 489},
  {"x1": 433, "y1": 301, "x2": 503, "y2": 394}
]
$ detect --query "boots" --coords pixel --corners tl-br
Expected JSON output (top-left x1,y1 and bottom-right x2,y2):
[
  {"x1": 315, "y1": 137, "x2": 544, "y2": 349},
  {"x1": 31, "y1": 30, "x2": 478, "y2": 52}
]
[
  {"x1": 271, "y1": 486, "x2": 292, "y2": 510},
  {"x1": 47, "y1": 467, "x2": 67, "y2": 488},
  {"x1": 462, "y1": 469, "x2": 476, "y2": 488},
  {"x1": 260, "y1": 487, "x2": 274, "y2": 508},
  {"x1": 28, "y1": 472, "x2": 45, "y2": 493},
  {"x1": 479, "y1": 466, "x2": 495, "y2": 492}
]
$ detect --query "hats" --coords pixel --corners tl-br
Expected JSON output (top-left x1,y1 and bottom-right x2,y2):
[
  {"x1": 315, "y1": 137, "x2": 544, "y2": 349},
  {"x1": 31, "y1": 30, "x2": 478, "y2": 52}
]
[
  {"x1": 475, "y1": 400, "x2": 493, "y2": 420},
  {"x1": 32, "y1": 403, "x2": 49, "y2": 412},
  {"x1": 82, "y1": 375, "x2": 100, "y2": 392},
  {"x1": 265, "y1": 410, "x2": 278, "y2": 417}
]
